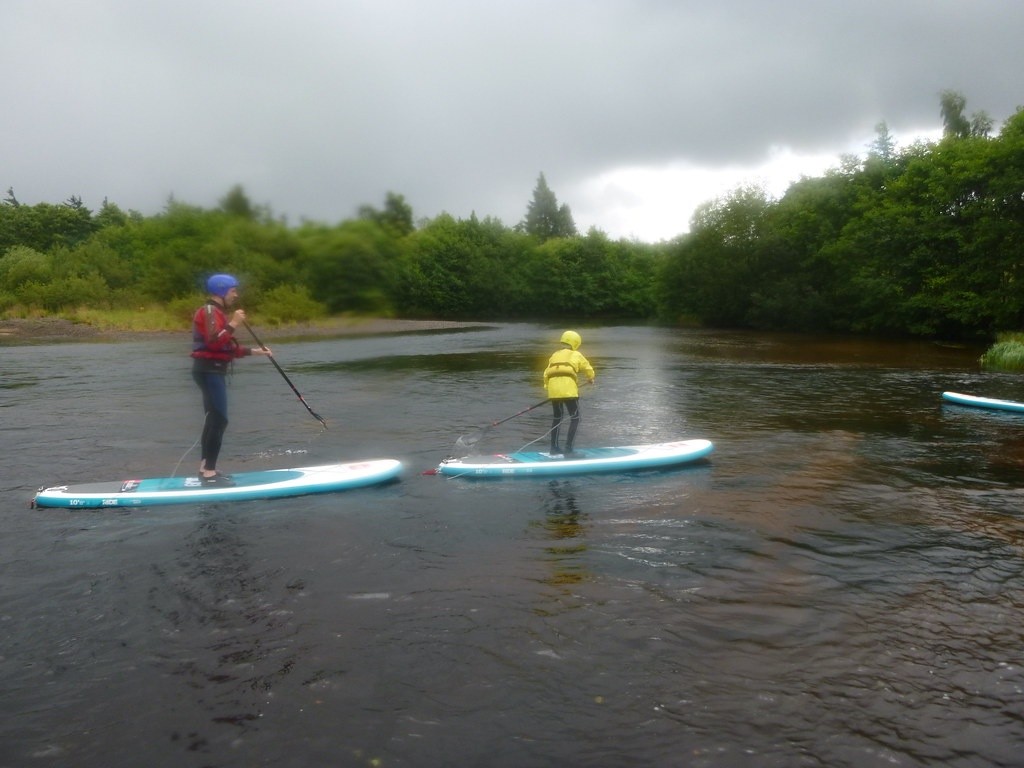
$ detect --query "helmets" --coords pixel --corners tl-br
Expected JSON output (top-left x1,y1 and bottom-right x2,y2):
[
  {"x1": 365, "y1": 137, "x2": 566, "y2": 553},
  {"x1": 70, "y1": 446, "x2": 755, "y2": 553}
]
[
  {"x1": 207, "y1": 274, "x2": 238, "y2": 298},
  {"x1": 560, "y1": 331, "x2": 582, "y2": 351}
]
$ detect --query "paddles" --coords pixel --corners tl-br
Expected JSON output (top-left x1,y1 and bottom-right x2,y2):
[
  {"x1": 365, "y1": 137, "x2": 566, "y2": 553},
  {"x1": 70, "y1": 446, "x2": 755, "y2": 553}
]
[
  {"x1": 463, "y1": 381, "x2": 589, "y2": 445},
  {"x1": 241, "y1": 318, "x2": 330, "y2": 431}
]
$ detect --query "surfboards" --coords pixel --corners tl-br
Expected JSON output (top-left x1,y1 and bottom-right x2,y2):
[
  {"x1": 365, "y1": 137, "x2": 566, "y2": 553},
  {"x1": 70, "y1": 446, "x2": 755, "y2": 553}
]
[
  {"x1": 33, "y1": 458, "x2": 405, "y2": 509},
  {"x1": 437, "y1": 436, "x2": 715, "y2": 479},
  {"x1": 941, "y1": 390, "x2": 1024, "y2": 413}
]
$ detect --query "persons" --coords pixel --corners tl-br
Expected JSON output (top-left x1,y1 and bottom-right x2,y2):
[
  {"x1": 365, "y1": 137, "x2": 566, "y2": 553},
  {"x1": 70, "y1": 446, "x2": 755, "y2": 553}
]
[
  {"x1": 190, "y1": 275, "x2": 272, "y2": 487},
  {"x1": 543, "y1": 330, "x2": 595, "y2": 460}
]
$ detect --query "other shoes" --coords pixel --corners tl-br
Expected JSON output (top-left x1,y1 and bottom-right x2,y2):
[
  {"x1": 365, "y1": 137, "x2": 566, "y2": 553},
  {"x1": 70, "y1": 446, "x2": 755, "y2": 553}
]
[
  {"x1": 550, "y1": 447, "x2": 565, "y2": 455},
  {"x1": 564, "y1": 451, "x2": 585, "y2": 458}
]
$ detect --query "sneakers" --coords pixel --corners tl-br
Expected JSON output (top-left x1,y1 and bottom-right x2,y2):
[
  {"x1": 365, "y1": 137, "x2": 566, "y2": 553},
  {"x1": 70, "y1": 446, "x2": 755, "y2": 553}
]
[{"x1": 198, "y1": 469, "x2": 237, "y2": 488}]
